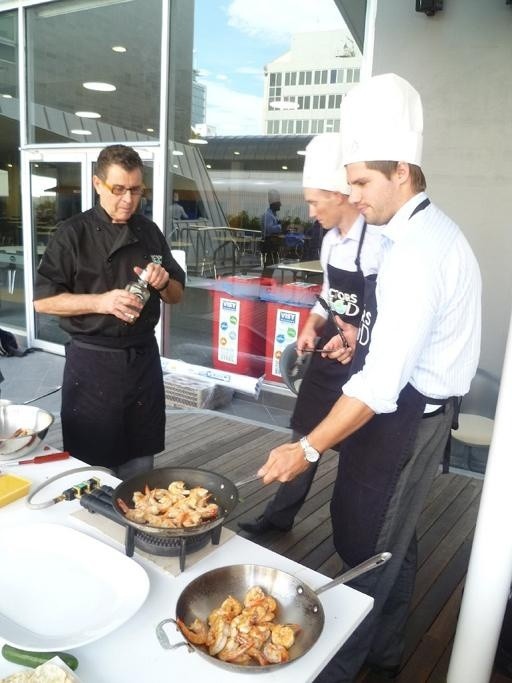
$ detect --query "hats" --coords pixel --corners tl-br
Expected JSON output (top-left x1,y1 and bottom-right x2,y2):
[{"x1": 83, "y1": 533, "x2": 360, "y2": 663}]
[
  {"x1": 302, "y1": 131, "x2": 351, "y2": 196},
  {"x1": 268, "y1": 190, "x2": 280, "y2": 205},
  {"x1": 340, "y1": 72, "x2": 424, "y2": 167}
]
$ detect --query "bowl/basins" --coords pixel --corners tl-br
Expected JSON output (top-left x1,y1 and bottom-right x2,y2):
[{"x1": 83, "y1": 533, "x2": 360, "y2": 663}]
[{"x1": 0, "y1": 403, "x2": 54, "y2": 461}]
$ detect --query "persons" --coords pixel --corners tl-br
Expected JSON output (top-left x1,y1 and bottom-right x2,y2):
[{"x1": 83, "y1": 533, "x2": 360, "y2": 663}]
[
  {"x1": 167, "y1": 188, "x2": 188, "y2": 240},
  {"x1": 32, "y1": 142, "x2": 185, "y2": 489},
  {"x1": 259, "y1": 158, "x2": 480, "y2": 683},
  {"x1": 261, "y1": 187, "x2": 292, "y2": 281},
  {"x1": 234, "y1": 184, "x2": 382, "y2": 535}
]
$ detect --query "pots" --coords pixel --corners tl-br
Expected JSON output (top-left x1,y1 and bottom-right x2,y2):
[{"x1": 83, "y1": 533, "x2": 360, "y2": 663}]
[
  {"x1": 156, "y1": 552, "x2": 395, "y2": 676},
  {"x1": 110, "y1": 469, "x2": 263, "y2": 538}
]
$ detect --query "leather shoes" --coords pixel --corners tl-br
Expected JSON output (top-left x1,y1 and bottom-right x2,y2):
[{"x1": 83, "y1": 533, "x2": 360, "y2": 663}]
[{"x1": 237, "y1": 512, "x2": 293, "y2": 532}]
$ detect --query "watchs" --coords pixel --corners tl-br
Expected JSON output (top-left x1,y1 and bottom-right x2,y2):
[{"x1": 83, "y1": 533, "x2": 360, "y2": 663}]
[{"x1": 299, "y1": 435, "x2": 321, "y2": 465}]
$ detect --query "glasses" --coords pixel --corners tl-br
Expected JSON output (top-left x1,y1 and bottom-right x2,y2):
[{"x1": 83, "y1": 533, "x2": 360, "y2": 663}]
[{"x1": 95, "y1": 175, "x2": 148, "y2": 195}]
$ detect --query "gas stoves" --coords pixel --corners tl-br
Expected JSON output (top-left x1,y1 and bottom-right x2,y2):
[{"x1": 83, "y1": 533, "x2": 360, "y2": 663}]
[{"x1": 77, "y1": 485, "x2": 226, "y2": 572}]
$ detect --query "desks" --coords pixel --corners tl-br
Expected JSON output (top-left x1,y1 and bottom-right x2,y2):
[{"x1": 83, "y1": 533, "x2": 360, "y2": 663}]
[{"x1": 0, "y1": 214, "x2": 323, "y2": 293}]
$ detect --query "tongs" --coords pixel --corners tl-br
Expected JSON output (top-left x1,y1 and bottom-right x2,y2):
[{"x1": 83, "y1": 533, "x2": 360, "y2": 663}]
[{"x1": 294, "y1": 294, "x2": 350, "y2": 355}]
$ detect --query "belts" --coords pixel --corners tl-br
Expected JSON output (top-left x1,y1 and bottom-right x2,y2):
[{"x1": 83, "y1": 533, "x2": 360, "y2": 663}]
[{"x1": 422, "y1": 406, "x2": 445, "y2": 418}]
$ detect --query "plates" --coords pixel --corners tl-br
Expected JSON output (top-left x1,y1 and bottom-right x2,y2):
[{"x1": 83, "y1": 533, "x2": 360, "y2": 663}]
[{"x1": 0, "y1": 507, "x2": 150, "y2": 655}]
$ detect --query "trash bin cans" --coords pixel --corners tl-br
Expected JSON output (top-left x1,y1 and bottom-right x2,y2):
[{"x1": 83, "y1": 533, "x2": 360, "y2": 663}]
[
  {"x1": 210, "y1": 274, "x2": 277, "y2": 378},
  {"x1": 265, "y1": 282, "x2": 321, "y2": 384}
]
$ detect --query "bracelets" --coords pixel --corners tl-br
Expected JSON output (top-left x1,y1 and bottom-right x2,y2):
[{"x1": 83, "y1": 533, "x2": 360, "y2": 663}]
[{"x1": 157, "y1": 278, "x2": 170, "y2": 291}]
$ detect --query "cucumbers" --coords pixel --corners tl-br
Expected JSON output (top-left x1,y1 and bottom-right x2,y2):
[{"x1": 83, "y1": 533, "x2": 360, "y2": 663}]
[{"x1": 1, "y1": 644, "x2": 79, "y2": 672}]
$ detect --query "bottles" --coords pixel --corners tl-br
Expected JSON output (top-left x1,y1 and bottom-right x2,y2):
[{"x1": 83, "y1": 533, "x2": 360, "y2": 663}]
[{"x1": 116, "y1": 260, "x2": 154, "y2": 326}]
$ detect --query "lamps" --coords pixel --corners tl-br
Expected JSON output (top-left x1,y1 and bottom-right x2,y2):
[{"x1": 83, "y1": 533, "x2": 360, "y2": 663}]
[{"x1": 416, "y1": 0, "x2": 443, "y2": 16}]
